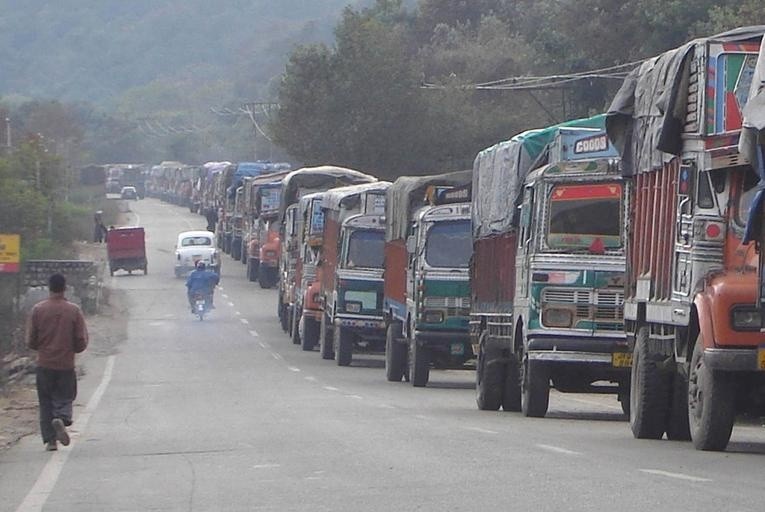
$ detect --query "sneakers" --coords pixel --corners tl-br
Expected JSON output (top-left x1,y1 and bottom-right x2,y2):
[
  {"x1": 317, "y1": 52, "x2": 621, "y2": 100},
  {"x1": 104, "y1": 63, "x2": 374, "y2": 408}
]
[
  {"x1": 47, "y1": 441, "x2": 57, "y2": 450},
  {"x1": 52, "y1": 419, "x2": 71, "y2": 447}
]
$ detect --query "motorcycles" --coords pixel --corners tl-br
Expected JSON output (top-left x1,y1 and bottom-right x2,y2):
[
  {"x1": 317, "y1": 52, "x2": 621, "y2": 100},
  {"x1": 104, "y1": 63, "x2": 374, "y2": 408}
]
[
  {"x1": 107, "y1": 226, "x2": 149, "y2": 276},
  {"x1": 195, "y1": 292, "x2": 210, "y2": 321}
]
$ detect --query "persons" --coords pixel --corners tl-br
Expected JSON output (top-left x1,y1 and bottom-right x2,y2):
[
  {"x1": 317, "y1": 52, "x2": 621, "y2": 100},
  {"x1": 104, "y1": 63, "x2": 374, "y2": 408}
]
[
  {"x1": 185, "y1": 259, "x2": 219, "y2": 315},
  {"x1": 26, "y1": 273, "x2": 89, "y2": 452},
  {"x1": 93, "y1": 210, "x2": 105, "y2": 243},
  {"x1": 206, "y1": 205, "x2": 218, "y2": 232}
]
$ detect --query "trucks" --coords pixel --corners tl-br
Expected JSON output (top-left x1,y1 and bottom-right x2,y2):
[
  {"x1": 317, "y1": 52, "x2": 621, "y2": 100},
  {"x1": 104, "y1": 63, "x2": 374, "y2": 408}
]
[
  {"x1": 106, "y1": 159, "x2": 381, "y2": 335},
  {"x1": 318, "y1": 181, "x2": 397, "y2": 366},
  {"x1": 469, "y1": 113, "x2": 636, "y2": 419},
  {"x1": 292, "y1": 192, "x2": 327, "y2": 351},
  {"x1": 380, "y1": 169, "x2": 479, "y2": 387},
  {"x1": 603, "y1": 22, "x2": 764, "y2": 452}
]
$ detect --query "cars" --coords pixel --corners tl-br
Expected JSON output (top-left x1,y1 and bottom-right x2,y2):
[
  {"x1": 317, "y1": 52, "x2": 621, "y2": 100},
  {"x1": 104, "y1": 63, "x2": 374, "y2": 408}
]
[
  {"x1": 120, "y1": 187, "x2": 138, "y2": 200},
  {"x1": 174, "y1": 231, "x2": 222, "y2": 277}
]
